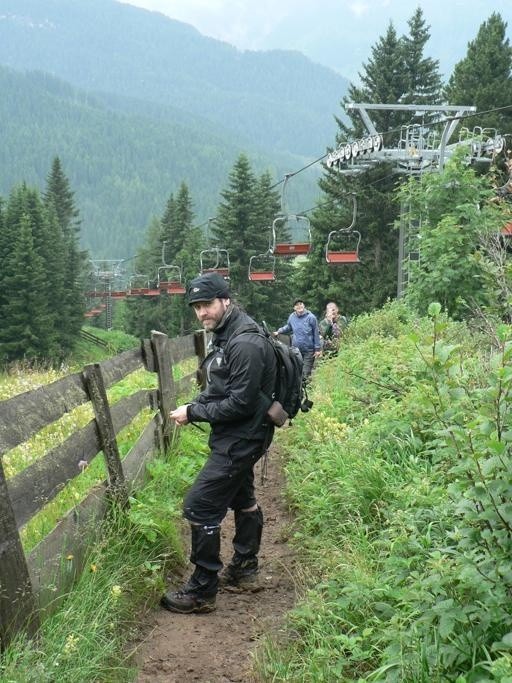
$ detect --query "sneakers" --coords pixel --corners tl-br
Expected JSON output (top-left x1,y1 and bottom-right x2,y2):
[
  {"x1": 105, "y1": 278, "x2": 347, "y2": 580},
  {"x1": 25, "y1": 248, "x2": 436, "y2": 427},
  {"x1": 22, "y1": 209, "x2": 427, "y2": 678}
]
[
  {"x1": 160, "y1": 586, "x2": 217, "y2": 614},
  {"x1": 217, "y1": 567, "x2": 265, "y2": 593}
]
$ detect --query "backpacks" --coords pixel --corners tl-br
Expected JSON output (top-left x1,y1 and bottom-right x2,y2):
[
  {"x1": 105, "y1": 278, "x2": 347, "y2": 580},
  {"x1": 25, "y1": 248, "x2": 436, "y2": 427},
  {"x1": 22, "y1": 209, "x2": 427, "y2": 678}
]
[{"x1": 227, "y1": 319, "x2": 306, "y2": 421}]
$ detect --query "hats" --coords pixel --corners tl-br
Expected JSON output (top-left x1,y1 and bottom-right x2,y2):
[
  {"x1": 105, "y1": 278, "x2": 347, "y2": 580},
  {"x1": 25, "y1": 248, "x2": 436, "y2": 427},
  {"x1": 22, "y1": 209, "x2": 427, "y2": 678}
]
[{"x1": 187, "y1": 271, "x2": 231, "y2": 307}]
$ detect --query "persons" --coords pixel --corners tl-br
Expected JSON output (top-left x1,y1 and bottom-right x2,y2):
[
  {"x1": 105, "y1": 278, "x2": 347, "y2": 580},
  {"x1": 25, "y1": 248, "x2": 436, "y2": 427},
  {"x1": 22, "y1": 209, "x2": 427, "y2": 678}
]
[
  {"x1": 158, "y1": 273, "x2": 279, "y2": 614},
  {"x1": 272, "y1": 297, "x2": 323, "y2": 384},
  {"x1": 317, "y1": 300, "x2": 347, "y2": 360}
]
